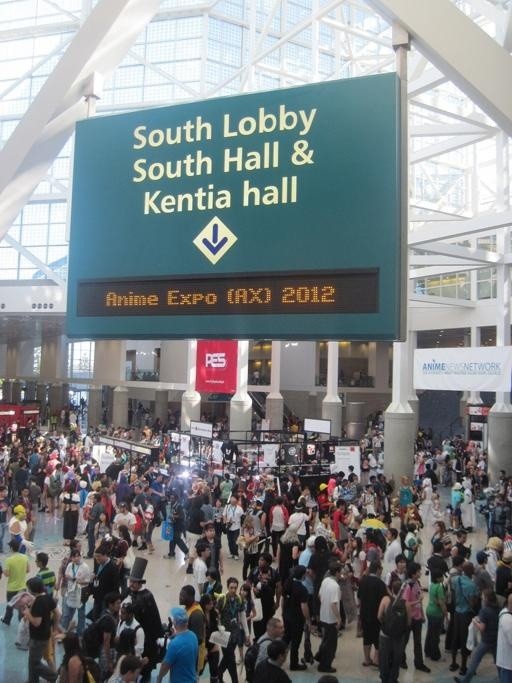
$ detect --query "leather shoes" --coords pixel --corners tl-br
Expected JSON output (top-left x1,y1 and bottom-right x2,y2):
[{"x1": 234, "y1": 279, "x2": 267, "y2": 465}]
[
  {"x1": 137, "y1": 545, "x2": 147, "y2": 550},
  {"x1": 399, "y1": 648, "x2": 477, "y2": 682},
  {"x1": 132, "y1": 541, "x2": 138, "y2": 547},
  {"x1": 290, "y1": 656, "x2": 337, "y2": 672},
  {"x1": 0, "y1": 617, "x2": 11, "y2": 626}
]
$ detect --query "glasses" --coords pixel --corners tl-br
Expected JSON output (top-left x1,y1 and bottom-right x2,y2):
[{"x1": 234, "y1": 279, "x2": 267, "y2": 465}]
[{"x1": 123, "y1": 603, "x2": 130, "y2": 613}]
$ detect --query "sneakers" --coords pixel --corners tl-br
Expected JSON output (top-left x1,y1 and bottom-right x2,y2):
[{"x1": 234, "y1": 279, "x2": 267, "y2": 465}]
[
  {"x1": 236, "y1": 657, "x2": 243, "y2": 665},
  {"x1": 184, "y1": 552, "x2": 189, "y2": 560},
  {"x1": 227, "y1": 554, "x2": 233, "y2": 558},
  {"x1": 163, "y1": 553, "x2": 175, "y2": 560},
  {"x1": 234, "y1": 555, "x2": 240, "y2": 560},
  {"x1": 83, "y1": 553, "x2": 92, "y2": 559}
]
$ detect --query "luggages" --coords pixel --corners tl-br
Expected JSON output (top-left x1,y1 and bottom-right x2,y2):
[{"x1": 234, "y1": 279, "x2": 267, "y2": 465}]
[{"x1": 236, "y1": 535, "x2": 249, "y2": 549}]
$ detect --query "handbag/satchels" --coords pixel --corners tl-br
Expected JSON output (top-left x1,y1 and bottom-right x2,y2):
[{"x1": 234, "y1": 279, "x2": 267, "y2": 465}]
[
  {"x1": 162, "y1": 515, "x2": 175, "y2": 540},
  {"x1": 157, "y1": 644, "x2": 166, "y2": 663},
  {"x1": 251, "y1": 586, "x2": 263, "y2": 622},
  {"x1": 222, "y1": 522, "x2": 227, "y2": 535},
  {"x1": 64, "y1": 580, "x2": 85, "y2": 609},
  {"x1": 445, "y1": 590, "x2": 455, "y2": 611},
  {"x1": 466, "y1": 617, "x2": 482, "y2": 651}
]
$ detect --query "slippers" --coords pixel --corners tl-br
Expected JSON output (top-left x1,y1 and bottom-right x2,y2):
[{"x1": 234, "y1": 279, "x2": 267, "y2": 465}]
[
  {"x1": 362, "y1": 658, "x2": 373, "y2": 667},
  {"x1": 62, "y1": 541, "x2": 70, "y2": 546},
  {"x1": 372, "y1": 658, "x2": 379, "y2": 666}
]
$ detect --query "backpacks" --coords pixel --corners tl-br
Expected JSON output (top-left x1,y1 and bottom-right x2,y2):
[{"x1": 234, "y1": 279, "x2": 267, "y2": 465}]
[
  {"x1": 244, "y1": 637, "x2": 273, "y2": 674},
  {"x1": 250, "y1": 510, "x2": 263, "y2": 537},
  {"x1": 149, "y1": 504, "x2": 161, "y2": 527},
  {"x1": 48, "y1": 475, "x2": 62, "y2": 497},
  {"x1": 78, "y1": 655, "x2": 100, "y2": 683},
  {"x1": 81, "y1": 614, "x2": 115, "y2": 660},
  {"x1": 380, "y1": 596, "x2": 407, "y2": 639}
]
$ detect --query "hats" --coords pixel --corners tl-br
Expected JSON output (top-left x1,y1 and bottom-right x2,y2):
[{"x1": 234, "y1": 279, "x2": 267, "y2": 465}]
[
  {"x1": 170, "y1": 608, "x2": 190, "y2": 626},
  {"x1": 364, "y1": 528, "x2": 373, "y2": 535},
  {"x1": 320, "y1": 483, "x2": 328, "y2": 492},
  {"x1": 5, "y1": 538, "x2": 22, "y2": 548},
  {"x1": 124, "y1": 557, "x2": 149, "y2": 584},
  {"x1": 117, "y1": 501, "x2": 128, "y2": 508},
  {"x1": 329, "y1": 562, "x2": 345, "y2": 574},
  {"x1": 451, "y1": 482, "x2": 463, "y2": 490},
  {"x1": 486, "y1": 536, "x2": 503, "y2": 551},
  {"x1": 293, "y1": 503, "x2": 305, "y2": 512}
]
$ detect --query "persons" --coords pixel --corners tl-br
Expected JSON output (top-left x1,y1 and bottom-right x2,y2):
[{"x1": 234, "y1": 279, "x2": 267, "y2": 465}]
[
  {"x1": 252, "y1": 368, "x2": 259, "y2": 383},
  {"x1": 0, "y1": 397, "x2": 512, "y2": 683},
  {"x1": 127, "y1": 367, "x2": 160, "y2": 381},
  {"x1": 337, "y1": 368, "x2": 373, "y2": 387}
]
